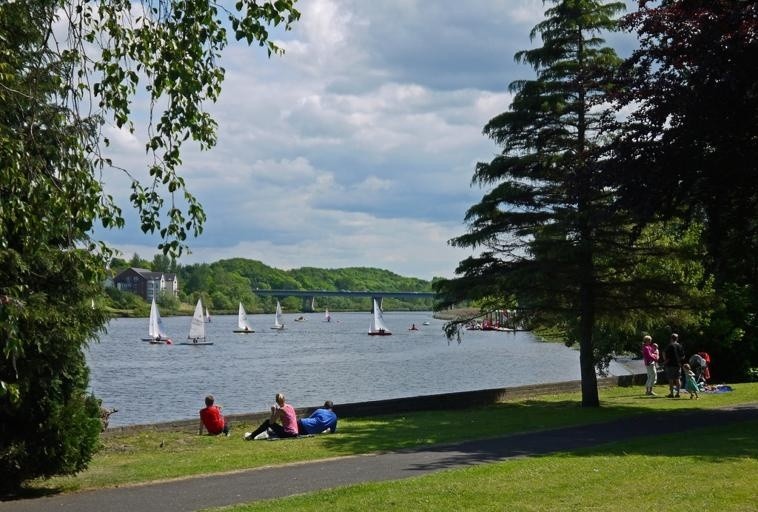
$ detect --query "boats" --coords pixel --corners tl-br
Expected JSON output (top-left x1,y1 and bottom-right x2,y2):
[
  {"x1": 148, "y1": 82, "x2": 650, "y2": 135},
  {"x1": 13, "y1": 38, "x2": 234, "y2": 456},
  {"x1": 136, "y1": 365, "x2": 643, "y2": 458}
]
[
  {"x1": 466, "y1": 321, "x2": 481, "y2": 330},
  {"x1": 409, "y1": 325, "x2": 419, "y2": 332},
  {"x1": 422, "y1": 321, "x2": 430, "y2": 325},
  {"x1": 295, "y1": 315, "x2": 306, "y2": 322}
]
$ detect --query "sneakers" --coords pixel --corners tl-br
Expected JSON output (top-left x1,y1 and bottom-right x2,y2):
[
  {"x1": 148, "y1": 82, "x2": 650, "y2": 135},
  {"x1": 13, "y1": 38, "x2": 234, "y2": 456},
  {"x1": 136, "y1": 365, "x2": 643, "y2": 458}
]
[
  {"x1": 267, "y1": 434, "x2": 280, "y2": 440},
  {"x1": 244, "y1": 436, "x2": 254, "y2": 440}
]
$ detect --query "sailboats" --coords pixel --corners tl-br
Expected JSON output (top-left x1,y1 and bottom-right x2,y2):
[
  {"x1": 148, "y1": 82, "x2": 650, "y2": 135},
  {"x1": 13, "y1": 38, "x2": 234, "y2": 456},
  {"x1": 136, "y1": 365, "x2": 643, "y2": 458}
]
[
  {"x1": 233, "y1": 300, "x2": 256, "y2": 334},
  {"x1": 270, "y1": 301, "x2": 284, "y2": 330},
  {"x1": 140, "y1": 295, "x2": 216, "y2": 347},
  {"x1": 367, "y1": 298, "x2": 392, "y2": 336},
  {"x1": 322, "y1": 307, "x2": 340, "y2": 324}
]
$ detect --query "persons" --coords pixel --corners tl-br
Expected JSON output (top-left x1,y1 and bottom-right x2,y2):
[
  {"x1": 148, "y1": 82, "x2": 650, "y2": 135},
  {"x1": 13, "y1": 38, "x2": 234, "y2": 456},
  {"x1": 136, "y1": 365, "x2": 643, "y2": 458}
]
[
  {"x1": 642, "y1": 333, "x2": 712, "y2": 399},
  {"x1": 199, "y1": 395, "x2": 231, "y2": 437},
  {"x1": 297, "y1": 401, "x2": 337, "y2": 435},
  {"x1": 193, "y1": 337, "x2": 199, "y2": 343},
  {"x1": 245, "y1": 326, "x2": 248, "y2": 330},
  {"x1": 156, "y1": 334, "x2": 161, "y2": 341},
  {"x1": 245, "y1": 393, "x2": 299, "y2": 441}
]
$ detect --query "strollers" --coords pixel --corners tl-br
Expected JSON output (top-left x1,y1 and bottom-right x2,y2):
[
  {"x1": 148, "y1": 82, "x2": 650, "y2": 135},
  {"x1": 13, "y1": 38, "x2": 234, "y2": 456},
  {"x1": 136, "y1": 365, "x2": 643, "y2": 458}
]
[{"x1": 684, "y1": 352, "x2": 711, "y2": 391}]
